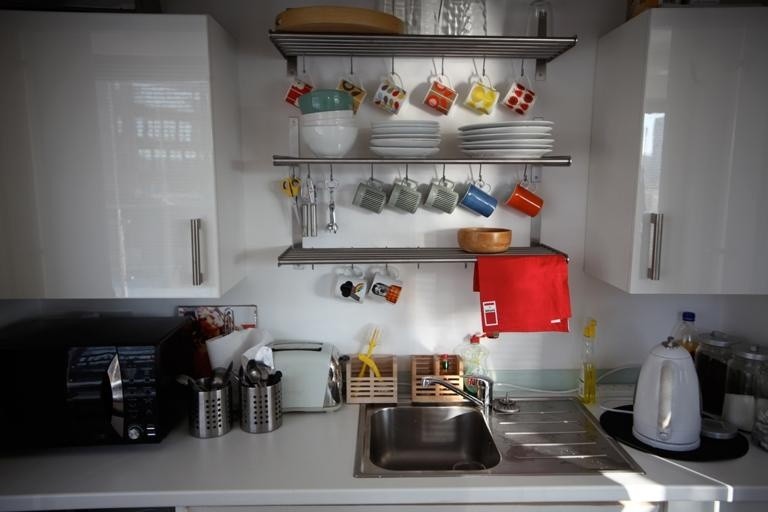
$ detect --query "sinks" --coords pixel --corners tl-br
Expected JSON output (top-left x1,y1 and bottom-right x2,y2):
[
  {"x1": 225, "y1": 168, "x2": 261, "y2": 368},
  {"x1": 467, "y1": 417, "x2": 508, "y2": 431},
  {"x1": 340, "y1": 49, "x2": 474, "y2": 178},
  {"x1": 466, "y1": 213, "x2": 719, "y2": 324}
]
[{"x1": 352, "y1": 399, "x2": 502, "y2": 476}]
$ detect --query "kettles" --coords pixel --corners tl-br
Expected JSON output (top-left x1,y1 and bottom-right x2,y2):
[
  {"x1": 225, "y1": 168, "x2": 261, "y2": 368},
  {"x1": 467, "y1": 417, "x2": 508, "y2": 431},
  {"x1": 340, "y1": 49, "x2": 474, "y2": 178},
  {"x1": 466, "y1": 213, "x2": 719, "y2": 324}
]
[{"x1": 630, "y1": 335, "x2": 708, "y2": 452}]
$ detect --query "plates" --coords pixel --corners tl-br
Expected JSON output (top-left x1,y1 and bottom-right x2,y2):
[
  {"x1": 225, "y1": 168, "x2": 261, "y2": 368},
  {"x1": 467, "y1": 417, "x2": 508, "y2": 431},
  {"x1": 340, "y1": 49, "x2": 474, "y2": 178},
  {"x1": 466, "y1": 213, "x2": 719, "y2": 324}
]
[
  {"x1": 599, "y1": 405, "x2": 751, "y2": 462},
  {"x1": 273, "y1": 4, "x2": 404, "y2": 33},
  {"x1": 368, "y1": 120, "x2": 443, "y2": 159},
  {"x1": 455, "y1": 119, "x2": 555, "y2": 161}
]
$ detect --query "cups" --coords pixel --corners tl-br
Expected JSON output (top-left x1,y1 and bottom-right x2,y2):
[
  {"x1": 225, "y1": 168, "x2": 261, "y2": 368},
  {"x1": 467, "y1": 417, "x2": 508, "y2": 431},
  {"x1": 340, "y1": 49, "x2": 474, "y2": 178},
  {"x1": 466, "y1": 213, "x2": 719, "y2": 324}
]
[
  {"x1": 351, "y1": 176, "x2": 389, "y2": 215},
  {"x1": 332, "y1": 265, "x2": 369, "y2": 305},
  {"x1": 503, "y1": 179, "x2": 543, "y2": 217},
  {"x1": 336, "y1": 73, "x2": 367, "y2": 114},
  {"x1": 283, "y1": 71, "x2": 315, "y2": 109},
  {"x1": 457, "y1": 181, "x2": 498, "y2": 217},
  {"x1": 421, "y1": 176, "x2": 460, "y2": 213},
  {"x1": 462, "y1": 74, "x2": 502, "y2": 116},
  {"x1": 422, "y1": 75, "x2": 460, "y2": 116},
  {"x1": 386, "y1": 178, "x2": 422, "y2": 215},
  {"x1": 371, "y1": 73, "x2": 409, "y2": 115},
  {"x1": 369, "y1": 265, "x2": 404, "y2": 305},
  {"x1": 501, "y1": 73, "x2": 540, "y2": 117}
]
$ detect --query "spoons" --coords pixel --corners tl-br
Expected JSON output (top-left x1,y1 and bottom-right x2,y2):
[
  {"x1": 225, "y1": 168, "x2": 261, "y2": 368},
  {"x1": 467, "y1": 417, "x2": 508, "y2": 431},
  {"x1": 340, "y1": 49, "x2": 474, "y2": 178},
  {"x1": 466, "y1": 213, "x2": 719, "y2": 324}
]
[
  {"x1": 249, "y1": 367, "x2": 262, "y2": 387},
  {"x1": 358, "y1": 355, "x2": 389, "y2": 392}
]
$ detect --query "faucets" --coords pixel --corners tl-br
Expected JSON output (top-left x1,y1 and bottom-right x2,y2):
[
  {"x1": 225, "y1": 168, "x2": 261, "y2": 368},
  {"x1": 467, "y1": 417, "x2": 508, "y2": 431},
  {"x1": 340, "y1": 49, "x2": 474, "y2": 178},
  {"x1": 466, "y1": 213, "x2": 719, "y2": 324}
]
[{"x1": 424, "y1": 373, "x2": 499, "y2": 415}]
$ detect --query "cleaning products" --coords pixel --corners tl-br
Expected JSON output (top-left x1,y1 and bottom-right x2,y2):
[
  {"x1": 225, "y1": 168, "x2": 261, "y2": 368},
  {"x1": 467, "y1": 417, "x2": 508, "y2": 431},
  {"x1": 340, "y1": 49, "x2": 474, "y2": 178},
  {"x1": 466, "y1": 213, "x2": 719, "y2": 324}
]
[
  {"x1": 579, "y1": 317, "x2": 597, "y2": 405},
  {"x1": 464, "y1": 332, "x2": 494, "y2": 392}
]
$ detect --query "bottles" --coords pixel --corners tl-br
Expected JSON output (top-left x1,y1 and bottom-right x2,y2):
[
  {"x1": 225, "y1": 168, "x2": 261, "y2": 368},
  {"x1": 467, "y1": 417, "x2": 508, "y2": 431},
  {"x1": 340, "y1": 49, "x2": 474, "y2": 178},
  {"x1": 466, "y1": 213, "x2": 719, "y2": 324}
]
[
  {"x1": 721, "y1": 347, "x2": 768, "y2": 432},
  {"x1": 694, "y1": 333, "x2": 733, "y2": 415},
  {"x1": 751, "y1": 363, "x2": 768, "y2": 453},
  {"x1": 674, "y1": 311, "x2": 698, "y2": 358}
]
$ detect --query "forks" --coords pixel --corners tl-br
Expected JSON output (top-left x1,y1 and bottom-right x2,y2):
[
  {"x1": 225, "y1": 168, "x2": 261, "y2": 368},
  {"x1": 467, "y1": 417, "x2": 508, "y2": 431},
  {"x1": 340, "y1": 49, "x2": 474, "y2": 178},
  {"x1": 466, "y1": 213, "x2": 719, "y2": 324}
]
[{"x1": 354, "y1": 327, "x2": 381, "y2": 397}]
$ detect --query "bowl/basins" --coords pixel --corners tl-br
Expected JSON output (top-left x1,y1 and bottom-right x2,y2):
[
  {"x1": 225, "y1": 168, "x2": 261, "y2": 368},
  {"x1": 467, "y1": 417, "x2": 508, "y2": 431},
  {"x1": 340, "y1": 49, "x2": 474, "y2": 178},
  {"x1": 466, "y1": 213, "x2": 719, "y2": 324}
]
[
  {"x1": 457, "y1": 226, "x2": 512, "y2": 254},
  {"x1": 295, "y1": 89, "x2": 361, "y2": 158}
]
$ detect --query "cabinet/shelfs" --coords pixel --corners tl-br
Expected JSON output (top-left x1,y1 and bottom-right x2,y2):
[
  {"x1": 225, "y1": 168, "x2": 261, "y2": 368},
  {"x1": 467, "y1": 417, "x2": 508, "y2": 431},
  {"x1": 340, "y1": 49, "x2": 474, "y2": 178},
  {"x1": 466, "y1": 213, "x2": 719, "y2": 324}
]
[
  {"x1": 0, "y1": 9, "x2": 245, "y2": 297},
  {"x1": 583, "y1": 5, "x2": 768, "y2": 295},
  {"x1": 266, "y1": 0, "x2": 573, "y2": 267},
  {"x1": 0, "y1": 391, "x2": 768, "y2": 512}
]
